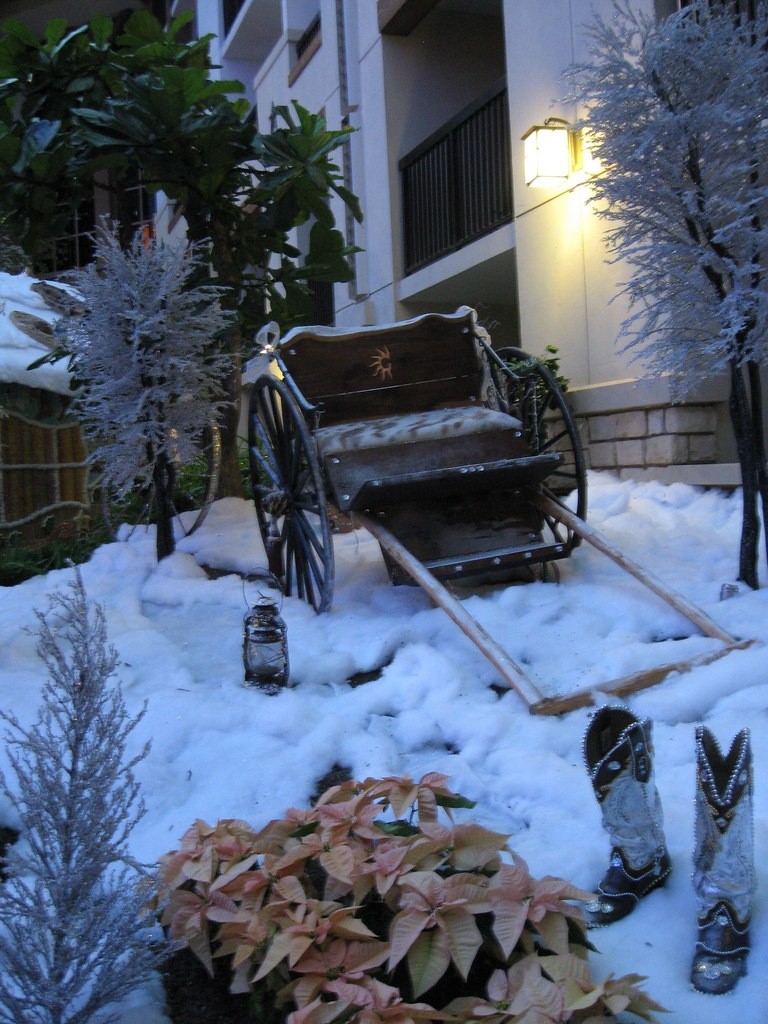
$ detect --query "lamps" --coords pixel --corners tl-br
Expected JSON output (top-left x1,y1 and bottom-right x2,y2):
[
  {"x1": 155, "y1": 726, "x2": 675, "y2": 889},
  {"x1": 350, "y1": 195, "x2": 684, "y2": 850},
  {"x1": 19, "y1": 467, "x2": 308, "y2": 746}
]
[
  {"x1": 521, "y1": 116, "x2": 577, "y2": 185},
  {"x1": 241, "y1": 566, "x2": 291, "y2": 693}
]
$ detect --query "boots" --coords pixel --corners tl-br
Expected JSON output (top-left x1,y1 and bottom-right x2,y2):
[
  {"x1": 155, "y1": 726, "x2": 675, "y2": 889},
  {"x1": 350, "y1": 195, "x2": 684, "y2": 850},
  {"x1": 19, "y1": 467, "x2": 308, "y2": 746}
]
[
  {"x1": 565, "y1": 704, "x2": 673, "y2": 928},
  {"x1": 691, "y1": 725, "x2": 757, "y2": 994}
]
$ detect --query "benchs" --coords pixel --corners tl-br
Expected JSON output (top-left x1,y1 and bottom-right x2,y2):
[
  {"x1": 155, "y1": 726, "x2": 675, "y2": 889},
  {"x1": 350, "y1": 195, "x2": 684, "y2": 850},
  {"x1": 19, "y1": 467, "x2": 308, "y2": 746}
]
[{"x1": 274, "y1": 304, "x2": 528, "y2": 503}]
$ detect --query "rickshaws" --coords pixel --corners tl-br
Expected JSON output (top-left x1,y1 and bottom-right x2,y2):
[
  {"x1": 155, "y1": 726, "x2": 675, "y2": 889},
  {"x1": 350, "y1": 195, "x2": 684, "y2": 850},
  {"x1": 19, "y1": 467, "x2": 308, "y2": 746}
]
[{"x1": 241, "y1": 305, "x2": 754, "y2": 714}]
